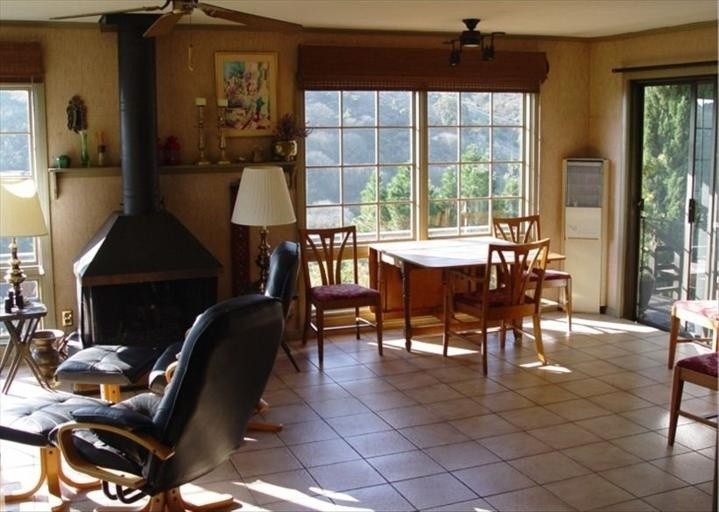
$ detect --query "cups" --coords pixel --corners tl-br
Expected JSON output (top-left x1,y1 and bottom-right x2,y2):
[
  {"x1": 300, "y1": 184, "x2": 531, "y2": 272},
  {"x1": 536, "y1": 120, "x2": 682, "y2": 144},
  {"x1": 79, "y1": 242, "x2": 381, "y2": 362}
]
[{"x1": 56, "y1": 156, "x2": 71, "y2": 168}]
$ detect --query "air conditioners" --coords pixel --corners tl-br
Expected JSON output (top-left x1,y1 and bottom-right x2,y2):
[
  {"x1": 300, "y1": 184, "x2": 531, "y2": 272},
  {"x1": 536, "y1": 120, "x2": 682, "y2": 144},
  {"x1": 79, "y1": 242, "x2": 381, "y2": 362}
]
[{"x1": 560, "y1": 155, "x2": 609, "y2": 316}]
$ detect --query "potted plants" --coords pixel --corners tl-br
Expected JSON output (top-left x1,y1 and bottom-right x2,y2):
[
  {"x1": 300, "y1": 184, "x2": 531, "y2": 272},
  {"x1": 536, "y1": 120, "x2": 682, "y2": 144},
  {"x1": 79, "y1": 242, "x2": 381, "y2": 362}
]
[{"x1": 274, "y1": 115, "x2": 298, "y2": 161}]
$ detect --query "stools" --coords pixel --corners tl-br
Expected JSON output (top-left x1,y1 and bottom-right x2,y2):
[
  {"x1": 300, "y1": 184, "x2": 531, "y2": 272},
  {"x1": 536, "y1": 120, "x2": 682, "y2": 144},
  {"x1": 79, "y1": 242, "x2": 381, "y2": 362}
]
[
  {"x1": 1, "y1": 391, "x2": 101, "y2": 512},
  {"x1": 55, "y1": 344, "x2": 157, "y2": 402},
  {"x1": 32, "y1": 329, "x2": 65, "y2": 380}
]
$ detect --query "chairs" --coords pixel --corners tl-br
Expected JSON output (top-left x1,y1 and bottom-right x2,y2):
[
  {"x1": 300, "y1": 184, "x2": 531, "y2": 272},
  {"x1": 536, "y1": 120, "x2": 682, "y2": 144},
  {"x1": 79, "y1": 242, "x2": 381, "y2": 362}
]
[
  {"x1": 299, "y1": 225, "x2": 383, "y2": 362},
  {"x1": 665, "y1": 299, "x2": 717, "y2": 371},
  {"x1": 490, "y1": 214, "x2": 573, "y2": 332},
  {"x1": 665, "y1": 353, "x2": 719, "y2": 447},
  {"x1": 442, "y1": 237, "x2": 551, "y2": 375},
  {"x1": 151, "y1": 241, "x2": 303, "y2": 436},
  {"x1": 498, "y1": 264, "x2": 573, "y2": 334},
  {"x1": 47, "y1": 296, "x2": 282, "y2": 512}
]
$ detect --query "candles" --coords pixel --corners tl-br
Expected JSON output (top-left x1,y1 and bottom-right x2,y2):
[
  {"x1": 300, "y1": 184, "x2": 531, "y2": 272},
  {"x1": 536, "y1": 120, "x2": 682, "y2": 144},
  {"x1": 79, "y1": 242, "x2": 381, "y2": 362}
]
[
  {"x1": 194, "y1": 97, "x2": 207, "y2": 105},
  {"x1": 216, "y1": 99, "x2": 228, "y2": 107}
]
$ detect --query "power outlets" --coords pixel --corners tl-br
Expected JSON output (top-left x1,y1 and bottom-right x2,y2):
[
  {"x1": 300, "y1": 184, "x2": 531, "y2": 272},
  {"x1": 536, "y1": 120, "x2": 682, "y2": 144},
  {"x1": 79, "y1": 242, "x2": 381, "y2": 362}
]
[{"x1": 62, "y1": 310, "x2": 74, "y2": 327}]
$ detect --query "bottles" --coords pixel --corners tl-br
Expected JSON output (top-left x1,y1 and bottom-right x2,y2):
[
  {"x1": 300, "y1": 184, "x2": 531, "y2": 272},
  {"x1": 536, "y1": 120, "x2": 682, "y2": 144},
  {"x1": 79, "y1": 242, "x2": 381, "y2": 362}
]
[
  {"x1": 164, "y1": 136, "x2": 181, "y2": 166},
  {"x1": 5, "y1": 300, "x2": 12, "y2": 313},
  {"x1": 8, "y1": 291, "x2": 13, "y2": 307},
  {"x1": 98, "y1": 145, "x2": 106, "y2": 166},
  {"x1": 251, "y1": 143, "x2": 265, "y2": 163}
]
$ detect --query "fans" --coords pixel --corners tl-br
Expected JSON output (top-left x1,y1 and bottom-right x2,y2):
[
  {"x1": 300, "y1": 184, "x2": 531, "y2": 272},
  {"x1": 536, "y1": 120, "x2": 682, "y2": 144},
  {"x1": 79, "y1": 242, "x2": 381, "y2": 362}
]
[{"x1": 50, "y1": 1, "x2": 311, "y2": 40}]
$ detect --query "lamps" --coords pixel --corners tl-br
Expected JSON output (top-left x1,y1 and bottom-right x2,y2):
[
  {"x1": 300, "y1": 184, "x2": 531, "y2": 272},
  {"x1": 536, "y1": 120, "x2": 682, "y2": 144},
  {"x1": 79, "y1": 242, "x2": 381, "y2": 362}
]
[
  {"x1": 443, "y1": 18, "x2": 505, "y2": 67},
  {"x1": 0, "y1": 175, "x2": 48, "y2": 306},
  {"x1": 229, "y1": 166, "x2": 295, "y2": 277}
]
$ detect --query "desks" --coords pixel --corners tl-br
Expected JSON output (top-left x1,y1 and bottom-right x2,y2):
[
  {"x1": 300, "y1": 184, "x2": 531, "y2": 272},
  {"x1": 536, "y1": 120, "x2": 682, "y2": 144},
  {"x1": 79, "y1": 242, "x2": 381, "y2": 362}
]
[{"x1": 372, "y1": 236, "x2": 550, "y2": 350}]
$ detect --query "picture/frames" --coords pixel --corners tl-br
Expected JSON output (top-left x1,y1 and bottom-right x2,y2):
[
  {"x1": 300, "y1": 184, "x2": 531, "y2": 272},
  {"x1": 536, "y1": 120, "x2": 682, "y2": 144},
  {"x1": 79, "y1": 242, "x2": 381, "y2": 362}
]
[{"x1": 215, "y1": 50, "x2": 279, "y2": 135}]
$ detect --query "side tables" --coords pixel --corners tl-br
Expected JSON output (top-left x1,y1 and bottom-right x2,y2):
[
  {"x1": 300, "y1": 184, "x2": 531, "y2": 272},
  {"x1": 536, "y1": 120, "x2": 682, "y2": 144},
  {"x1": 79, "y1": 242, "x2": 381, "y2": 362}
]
[{"x1": 0, "y1": 301, "x2": 49, "y2": 394}]
